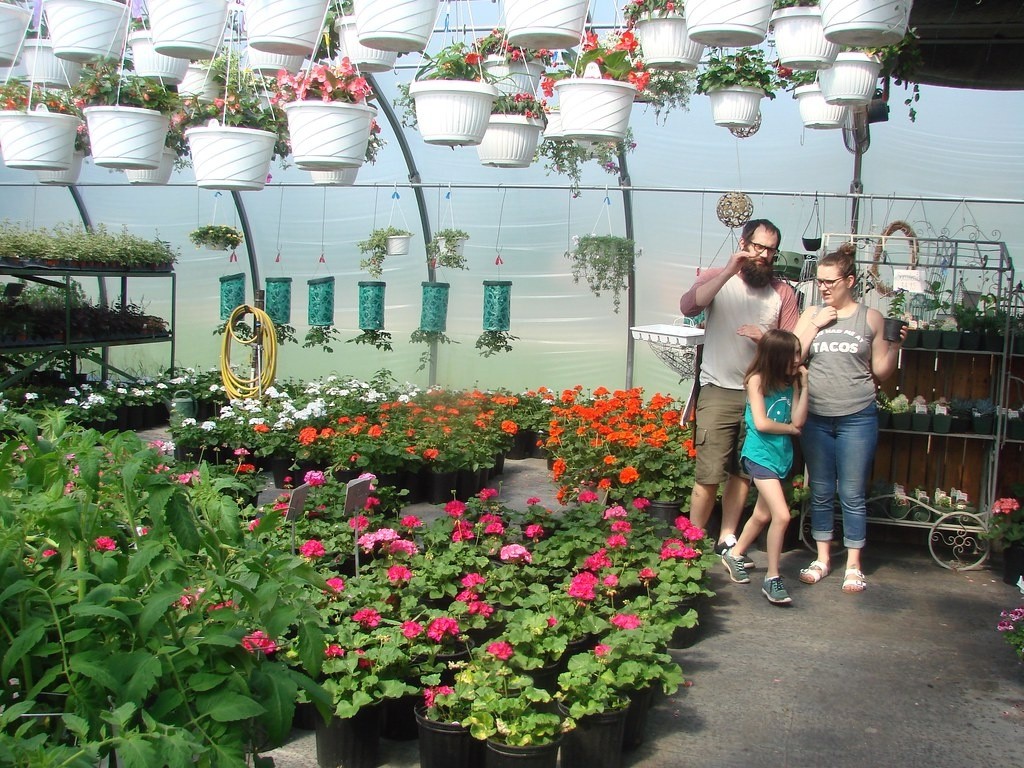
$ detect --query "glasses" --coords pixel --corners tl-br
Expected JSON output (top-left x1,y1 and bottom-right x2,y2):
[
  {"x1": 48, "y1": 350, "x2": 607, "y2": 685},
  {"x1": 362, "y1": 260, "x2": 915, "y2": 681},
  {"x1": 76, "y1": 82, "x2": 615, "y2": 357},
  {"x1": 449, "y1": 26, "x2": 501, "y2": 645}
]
[
  {"x1": 743, "y1": 237, "x2": 780, "y2": 253},
  {"x1": 814, "y1": 276, "x2": 847, "y2": 288}
]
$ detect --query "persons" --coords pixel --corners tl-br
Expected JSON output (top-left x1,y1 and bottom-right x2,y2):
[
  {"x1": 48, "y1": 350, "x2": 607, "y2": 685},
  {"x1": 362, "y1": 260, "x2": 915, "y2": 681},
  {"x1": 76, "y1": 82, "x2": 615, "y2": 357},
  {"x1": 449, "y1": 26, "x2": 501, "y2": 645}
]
[
  {"x1": 791, "y1": 245, "x2": 909, "y2": 592},
  {"x1": 680, "y1": 218, "x2": 799, "y2": 569},
  {"x1": 723, "y1": 328, "x2": 810, "y2": 604}
]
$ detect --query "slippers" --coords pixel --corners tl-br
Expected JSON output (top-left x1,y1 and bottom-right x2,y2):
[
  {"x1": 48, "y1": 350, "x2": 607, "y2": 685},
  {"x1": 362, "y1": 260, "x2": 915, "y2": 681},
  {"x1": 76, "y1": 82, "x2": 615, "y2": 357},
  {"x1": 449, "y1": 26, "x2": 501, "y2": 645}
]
[
  {"x1": 799, "y1": 561, "x2": 830, "y2": 583},
  {"x1": 842, "y1": 569, "x2": 864, "y2": 591}
]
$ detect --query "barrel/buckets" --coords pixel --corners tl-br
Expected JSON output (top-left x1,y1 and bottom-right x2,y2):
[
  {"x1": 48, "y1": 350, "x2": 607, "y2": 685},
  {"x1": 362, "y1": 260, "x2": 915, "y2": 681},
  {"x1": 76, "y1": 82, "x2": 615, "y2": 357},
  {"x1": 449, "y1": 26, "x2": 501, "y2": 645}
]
[{"x1": 962, "y1": 261, "x2": 984, "y2": 310}]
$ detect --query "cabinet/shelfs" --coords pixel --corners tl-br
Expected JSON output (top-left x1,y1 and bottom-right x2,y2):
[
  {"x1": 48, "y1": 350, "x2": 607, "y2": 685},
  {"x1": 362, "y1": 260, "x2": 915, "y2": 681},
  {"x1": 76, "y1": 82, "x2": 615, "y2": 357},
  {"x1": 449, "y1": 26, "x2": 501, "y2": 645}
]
[
  {"x1": 0, "y1": 266, "x2": 176, "y2": 388},
  {"x1": 797, "y1": 231, "x2": 1024, "y2": 573}
]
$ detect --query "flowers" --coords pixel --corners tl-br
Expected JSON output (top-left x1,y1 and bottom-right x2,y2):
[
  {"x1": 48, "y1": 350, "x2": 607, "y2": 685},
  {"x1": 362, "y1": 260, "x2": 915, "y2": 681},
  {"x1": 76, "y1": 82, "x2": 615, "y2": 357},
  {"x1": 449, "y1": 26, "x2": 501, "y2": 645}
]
[
  {"x1": 356, "y1": 227, "x2": 414, "y2": 278},
  {"x1": 189, "y1": 225, "x2": 245, "y2": 249},
  {"x1": 427, "y1": 229, "x2": 469, "y2": 270},
  {"x1": 570, "y1": 234, "x2": 643, "y2": 315},
  {"x1": 976, "y1": 482, "x2": 1024, "y2": 550},
  {"x1": 0, "y1": 0, "x2": 921, "y2": 197},
  {"x1": 779, "y1": 473, "x2": 809, "y2": 518},
  {"x1": 0, "y1": 364, "x2": 717, "y2": 746},
  {"x1": 992, "y1": 606, "x2": 1024, "y2": 664}
]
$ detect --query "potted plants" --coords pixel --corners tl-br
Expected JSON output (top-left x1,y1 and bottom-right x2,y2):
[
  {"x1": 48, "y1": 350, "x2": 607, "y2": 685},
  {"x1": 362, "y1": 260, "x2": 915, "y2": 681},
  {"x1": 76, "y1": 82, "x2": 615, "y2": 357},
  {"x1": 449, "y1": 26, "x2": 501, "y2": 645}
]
[
  {"x1": 871, "y1": 481, "x2": 975, "y2": 523},
  {"x1": 212, "y1": 273, "x2": 521, "y2": 372},
  {"x1": 0, "y1": 282, "x2": 171, "y2": 341},
  {"x1": 873, "y1": 389, "x2": 1024, "y2": 441},
  {"x1": 881, "y1": 281, "x2": 1024, "y2": 354},
  {"x1": 0, "y1": 216, "x2": 181, "y2": 271}
]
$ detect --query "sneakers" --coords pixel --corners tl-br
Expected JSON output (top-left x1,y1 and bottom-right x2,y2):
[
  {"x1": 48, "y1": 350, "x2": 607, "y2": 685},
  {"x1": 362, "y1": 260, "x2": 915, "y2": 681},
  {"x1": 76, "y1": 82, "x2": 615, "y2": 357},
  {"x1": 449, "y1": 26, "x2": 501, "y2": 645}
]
[
  {"x1": 714, "y1": 534, "x2": 754, "y2": 568},
  {"x1": 761, "y1": 575, "x2": 792, "y2": 602},
  {"x1": 721, "y1": 548, "x2": 751, "y2": 583}
]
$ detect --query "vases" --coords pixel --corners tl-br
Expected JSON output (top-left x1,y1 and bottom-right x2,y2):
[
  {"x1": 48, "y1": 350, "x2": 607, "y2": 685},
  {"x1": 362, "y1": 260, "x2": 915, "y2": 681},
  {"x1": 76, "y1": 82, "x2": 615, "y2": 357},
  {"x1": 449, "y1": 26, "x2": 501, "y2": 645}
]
[
  {"x1": 69, "y1": 374, "x2": 88, "y2": 384},
  {"x1": 77, "y1": 402, "x2": 794, "y2": 767},
  {"x1": 38, "y1": 371, "x2": 61, "y2": 386},
  {"x1": 991, "y1": 539, "x2": 1011, "y2": 573},
  {"x1": 0, "y1": 0, "x2": 912, "y2": 256},
  {"x1": 1003, "y1": 545, "x2": 1024, "y2": 587}
]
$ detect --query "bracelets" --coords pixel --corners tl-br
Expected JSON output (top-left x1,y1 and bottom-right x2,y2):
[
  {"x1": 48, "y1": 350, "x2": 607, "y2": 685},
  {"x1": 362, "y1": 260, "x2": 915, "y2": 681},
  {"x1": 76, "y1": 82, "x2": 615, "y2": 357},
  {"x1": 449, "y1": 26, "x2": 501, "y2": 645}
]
[{"x1": 811, "y1": 321, "x2": 822, "y2": 328}]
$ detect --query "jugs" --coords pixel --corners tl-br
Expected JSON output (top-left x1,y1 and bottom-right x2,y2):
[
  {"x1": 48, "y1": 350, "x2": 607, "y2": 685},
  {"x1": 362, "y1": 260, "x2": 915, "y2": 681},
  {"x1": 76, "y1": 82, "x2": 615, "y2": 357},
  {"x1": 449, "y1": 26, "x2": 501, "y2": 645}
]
[{"x1": 160, "y1": 390, "x2": 198, "y2": 427}]
